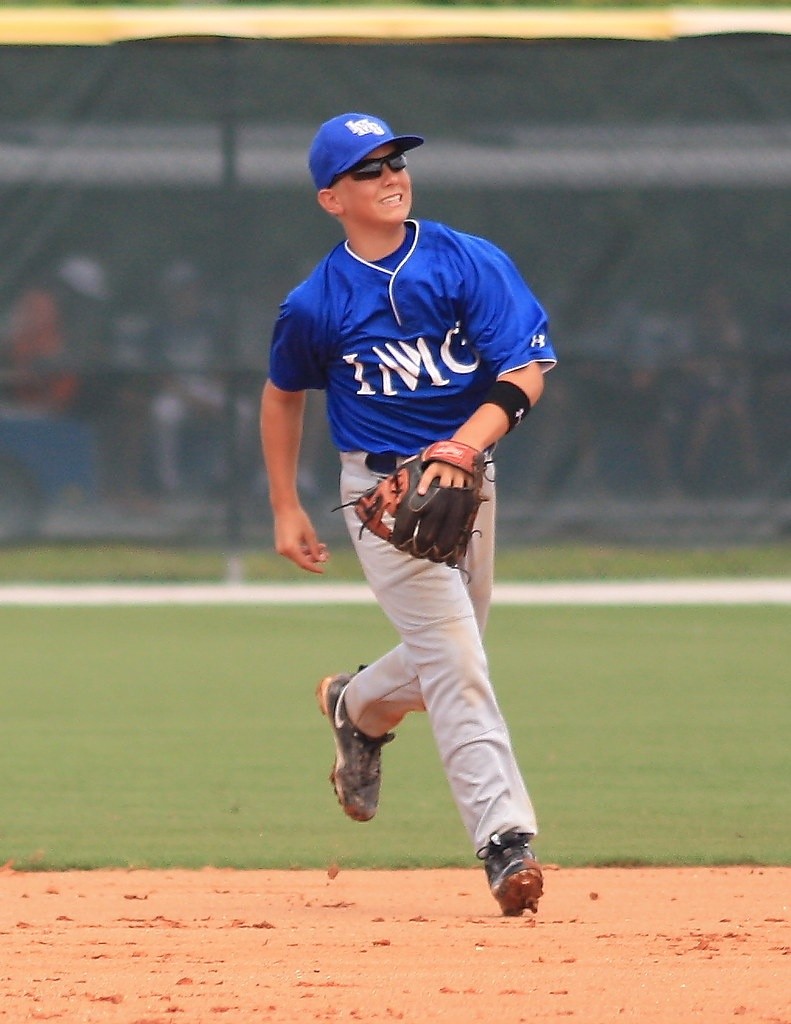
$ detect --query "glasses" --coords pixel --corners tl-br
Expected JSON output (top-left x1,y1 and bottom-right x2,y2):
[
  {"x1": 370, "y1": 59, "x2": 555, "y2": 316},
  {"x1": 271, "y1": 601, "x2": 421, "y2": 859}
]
[{"x1": 326, "y1": 148, "x2": 407, "y2": 189}]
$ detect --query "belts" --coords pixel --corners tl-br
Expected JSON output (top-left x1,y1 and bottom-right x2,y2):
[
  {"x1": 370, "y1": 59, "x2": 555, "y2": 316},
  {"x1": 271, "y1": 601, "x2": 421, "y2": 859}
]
[{"x1": 366, "y1": 445, "x2": 492, "y2": 477}]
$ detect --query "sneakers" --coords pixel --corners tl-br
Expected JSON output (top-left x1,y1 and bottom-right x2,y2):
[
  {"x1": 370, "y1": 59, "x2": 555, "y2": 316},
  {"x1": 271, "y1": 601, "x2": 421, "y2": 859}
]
[
  {"x1": 317, "y1": 664, "x2": 395, "y2": 822},
  {"x1": 476, "y1": 832, "x2": 544, "y2": 916}
]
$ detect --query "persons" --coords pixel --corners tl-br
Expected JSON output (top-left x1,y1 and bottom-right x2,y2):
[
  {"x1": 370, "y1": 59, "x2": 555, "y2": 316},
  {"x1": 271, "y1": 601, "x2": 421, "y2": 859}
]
[
  {"x1": 1, "y1": 256, "x2": 105, "y2": 535},
  {"x1": 151, "y1": 277, "x2": 226, "y2": 486},
  {"x1": 260, "y1": 113, "x2": 556, "y2": 918}
]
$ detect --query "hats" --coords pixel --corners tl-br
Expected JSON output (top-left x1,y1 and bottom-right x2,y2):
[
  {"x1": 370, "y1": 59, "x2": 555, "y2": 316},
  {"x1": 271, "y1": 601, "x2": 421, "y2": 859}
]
[{"x1": 308, "y1": 113, "x2": 424, "y2": 190}]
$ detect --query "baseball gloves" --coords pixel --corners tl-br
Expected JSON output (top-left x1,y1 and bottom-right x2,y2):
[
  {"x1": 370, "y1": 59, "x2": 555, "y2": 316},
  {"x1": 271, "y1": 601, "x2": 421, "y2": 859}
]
[{"x1": 350, "y1": 440, "x2": 489, "y2": 569}]
